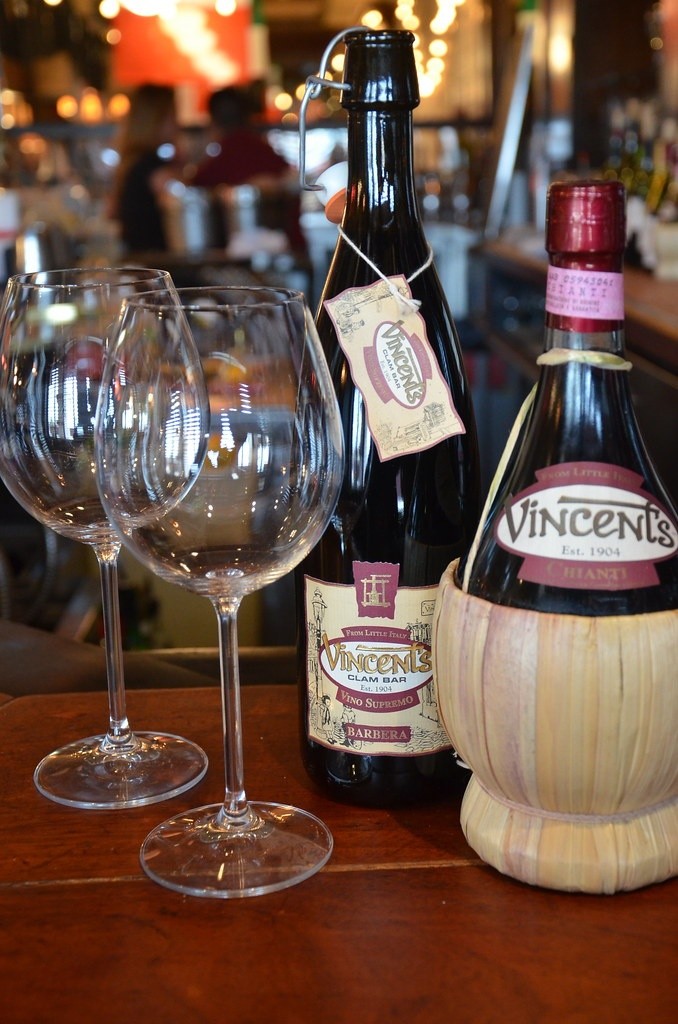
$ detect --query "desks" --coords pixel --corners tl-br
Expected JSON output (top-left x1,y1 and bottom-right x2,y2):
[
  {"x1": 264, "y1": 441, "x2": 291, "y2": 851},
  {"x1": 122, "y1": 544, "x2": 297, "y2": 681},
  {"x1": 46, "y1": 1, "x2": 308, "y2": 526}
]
[{"x1": 0, "y1": 690, "x2": 678, "y2": 1024}]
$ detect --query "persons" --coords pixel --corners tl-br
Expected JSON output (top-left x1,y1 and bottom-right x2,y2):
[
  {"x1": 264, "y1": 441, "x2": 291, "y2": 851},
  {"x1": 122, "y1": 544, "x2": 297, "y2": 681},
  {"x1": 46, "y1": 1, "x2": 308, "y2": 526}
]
[
  {"x1": 104, "y1": 86, "x2": 183, "y2": 259},
  {"x1": 182, "y1": 86, "x2": 314, "y2": 315}
]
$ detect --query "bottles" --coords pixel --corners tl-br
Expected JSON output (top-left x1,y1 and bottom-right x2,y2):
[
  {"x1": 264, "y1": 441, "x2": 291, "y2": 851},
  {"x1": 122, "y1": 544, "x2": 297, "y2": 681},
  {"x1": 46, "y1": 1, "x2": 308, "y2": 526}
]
[
  {"x1": 456, "y1": 180, "x2": 678, "y2": 616},
  {"x1": 299, "y1": 27, "x2": 471, "y2": 811}
]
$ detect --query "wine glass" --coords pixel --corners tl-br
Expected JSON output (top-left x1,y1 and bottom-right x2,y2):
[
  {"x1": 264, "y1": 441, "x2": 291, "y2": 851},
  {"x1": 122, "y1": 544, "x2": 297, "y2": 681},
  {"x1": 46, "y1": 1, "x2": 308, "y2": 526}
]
[
  {"x1": 89, "y1": 285, "x2": 340, "y2": 897},
  {"x1": 0, "y1": 268, "x2": 209, "y2": 810}
]
